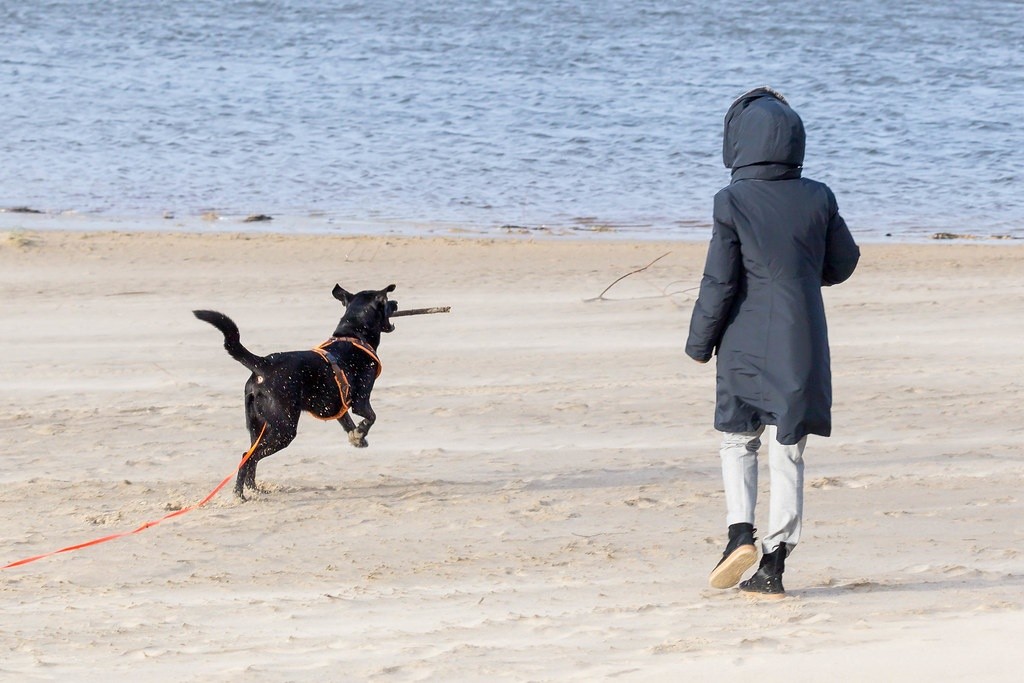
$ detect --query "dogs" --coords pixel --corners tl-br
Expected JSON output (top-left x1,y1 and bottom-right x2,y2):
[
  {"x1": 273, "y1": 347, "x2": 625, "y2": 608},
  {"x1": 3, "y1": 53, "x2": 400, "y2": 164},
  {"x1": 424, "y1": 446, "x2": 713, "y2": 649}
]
[{"x1": 191, "y1": 284, "x2": 398, "y2": 503}]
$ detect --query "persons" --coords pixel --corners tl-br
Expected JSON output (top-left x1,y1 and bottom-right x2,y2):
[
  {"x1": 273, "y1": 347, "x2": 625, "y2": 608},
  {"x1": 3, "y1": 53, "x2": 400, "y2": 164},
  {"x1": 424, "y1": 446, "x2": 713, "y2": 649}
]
[{"x1": 684, "y1": 87, "x2": 859, "y2": 599}]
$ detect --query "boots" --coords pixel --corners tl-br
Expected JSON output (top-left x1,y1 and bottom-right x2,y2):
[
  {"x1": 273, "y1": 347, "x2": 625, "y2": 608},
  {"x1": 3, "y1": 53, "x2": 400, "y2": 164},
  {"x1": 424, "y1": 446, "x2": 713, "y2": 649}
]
[
  {"x1": 740, "y1": 540, "x2": 789, "y2": 601},
  {"x1": 709, "y1": 522, "x2": 755, "y2": 590}
]
